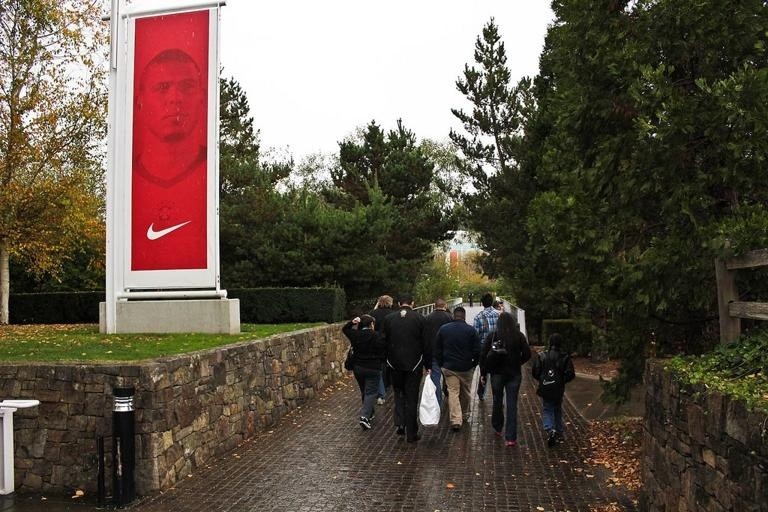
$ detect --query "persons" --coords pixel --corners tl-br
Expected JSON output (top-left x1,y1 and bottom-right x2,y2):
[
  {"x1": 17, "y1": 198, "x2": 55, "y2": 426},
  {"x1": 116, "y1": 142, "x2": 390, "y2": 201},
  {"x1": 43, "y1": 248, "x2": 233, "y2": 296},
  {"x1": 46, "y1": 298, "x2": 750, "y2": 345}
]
[
  {"x1": 133, "y1": 48, "x2": 207, "y2": 270},
  {"x1": 341, "y1": 291, "x2": 531, "y2": 447},
  {"x1": 532, "y1": 333, "x2": 575, "y2": 448}
]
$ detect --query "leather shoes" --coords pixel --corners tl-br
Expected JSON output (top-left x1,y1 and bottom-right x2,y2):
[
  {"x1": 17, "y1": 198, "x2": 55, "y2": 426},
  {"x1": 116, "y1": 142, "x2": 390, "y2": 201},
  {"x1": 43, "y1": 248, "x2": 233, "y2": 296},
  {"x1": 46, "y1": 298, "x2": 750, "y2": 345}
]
[
  {"x1": 407, "y1": 434, "x2": 421, "y2": 443},
  {"x1": 397, "y1": 424, "x2": 406, "y2": 435}
]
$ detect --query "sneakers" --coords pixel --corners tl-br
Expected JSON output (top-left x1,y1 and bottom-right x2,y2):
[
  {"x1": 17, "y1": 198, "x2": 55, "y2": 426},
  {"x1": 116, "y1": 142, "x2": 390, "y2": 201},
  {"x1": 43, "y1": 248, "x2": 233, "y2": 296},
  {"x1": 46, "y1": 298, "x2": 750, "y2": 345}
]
[
  {"x1": 496, "y1": 432, "x2": 504, "y2": 439},
  {"x1": 359, "y1": 416, "x2": 371, "y2": 430},
  {"x1": 377, "y1": 398, "x2": 385, "y2": 405},
  {"x1": 505, "y1": 440, "x2": 517, "y2": 447},
  {"x1": 547, "y1": 428, "x2": 565, "y2": 448},
  {"x1": 453, "y1": 423, "x2": 463, "y2": 432},
  {"x1": 368, "y1": 414, "x2": 375, "y2": 421}
]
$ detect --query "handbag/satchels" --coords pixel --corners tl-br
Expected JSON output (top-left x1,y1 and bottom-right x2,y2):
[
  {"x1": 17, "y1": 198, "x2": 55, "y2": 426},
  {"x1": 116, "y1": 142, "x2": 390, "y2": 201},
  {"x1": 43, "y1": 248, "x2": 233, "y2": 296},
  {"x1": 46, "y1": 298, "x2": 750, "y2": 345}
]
[
  {"x1": 483, "y1": 329, "x2": 509, "y2": 372},
  {"x1": 344, "y1": 345, "x2": 354, "y2": 371}
]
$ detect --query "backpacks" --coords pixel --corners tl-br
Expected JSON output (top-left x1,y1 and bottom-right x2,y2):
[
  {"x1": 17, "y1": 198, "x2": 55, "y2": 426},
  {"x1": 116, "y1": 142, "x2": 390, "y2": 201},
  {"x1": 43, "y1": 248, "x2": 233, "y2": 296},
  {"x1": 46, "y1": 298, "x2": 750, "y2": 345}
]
[{"x1": 536, "y1": 350, "x2": 565, "y2": 401}]
